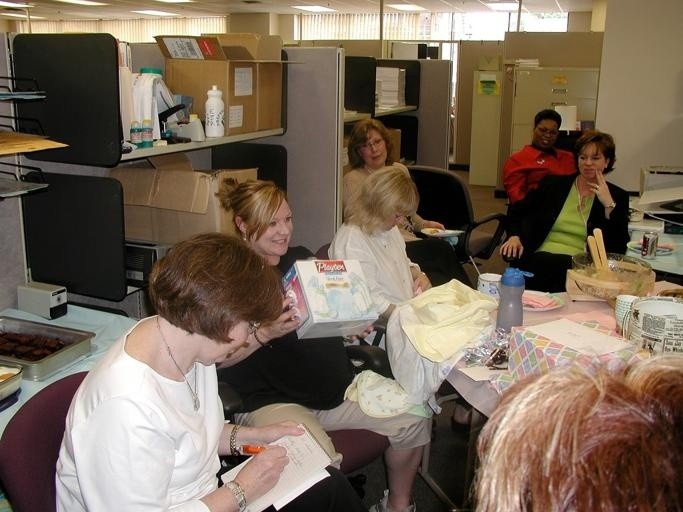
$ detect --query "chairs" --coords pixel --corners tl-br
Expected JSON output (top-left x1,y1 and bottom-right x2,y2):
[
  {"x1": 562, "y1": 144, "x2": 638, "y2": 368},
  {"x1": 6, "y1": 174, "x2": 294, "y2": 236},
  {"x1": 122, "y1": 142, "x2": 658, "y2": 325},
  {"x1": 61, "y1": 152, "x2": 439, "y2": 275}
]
[
  {"x1": 405, "y1": 165, "x2": 506, "y2": 267},
  {"x1": 0, "y1": 370, "x2": 89, "y2": 512},
  {"x1": 324, "y1": 345, "x2": 395, "y2": 499}
]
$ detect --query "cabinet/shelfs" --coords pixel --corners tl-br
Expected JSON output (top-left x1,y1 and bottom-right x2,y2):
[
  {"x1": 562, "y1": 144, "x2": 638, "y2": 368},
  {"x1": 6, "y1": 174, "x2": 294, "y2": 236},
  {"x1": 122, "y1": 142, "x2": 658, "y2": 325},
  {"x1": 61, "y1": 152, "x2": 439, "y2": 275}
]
[
  {"x1": 342, "y1": 57, "x2": 454, "y2": 227},
  {"x1": 509, "y1": 67, "x2": 600, "y2": 160},
  {"x1": 0, "y1": 32, "x2": 340, "y2": 321}
]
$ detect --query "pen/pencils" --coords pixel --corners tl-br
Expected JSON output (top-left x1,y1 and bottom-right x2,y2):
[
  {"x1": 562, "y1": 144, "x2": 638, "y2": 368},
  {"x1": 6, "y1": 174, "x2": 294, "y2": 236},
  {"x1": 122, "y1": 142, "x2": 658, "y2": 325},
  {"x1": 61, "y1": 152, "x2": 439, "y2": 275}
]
[{"x1": 236, "y1": 445, "x2": 265, "y2": 456}]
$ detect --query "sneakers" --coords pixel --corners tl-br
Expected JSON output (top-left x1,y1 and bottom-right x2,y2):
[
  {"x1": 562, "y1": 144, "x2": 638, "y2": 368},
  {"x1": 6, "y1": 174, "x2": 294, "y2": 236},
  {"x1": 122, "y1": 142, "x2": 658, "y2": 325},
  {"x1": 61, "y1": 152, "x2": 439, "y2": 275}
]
[{"x1": 369, "y1": 489, "x2": 417, "y2": 512}]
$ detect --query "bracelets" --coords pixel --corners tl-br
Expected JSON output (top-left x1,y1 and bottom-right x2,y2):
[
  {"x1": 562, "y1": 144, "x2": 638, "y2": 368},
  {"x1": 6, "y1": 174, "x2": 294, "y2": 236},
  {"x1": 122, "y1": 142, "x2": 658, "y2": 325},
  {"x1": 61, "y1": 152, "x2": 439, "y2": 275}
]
[
  {"x1": 230, "y1": 425, "x2": 241, "y2": 458},
  {"x1": 605, "y1": 201, "x2": 618, "y2": 209},
  {"x1": 225, "y1": 480, "x2": 247, "y2": 511}
]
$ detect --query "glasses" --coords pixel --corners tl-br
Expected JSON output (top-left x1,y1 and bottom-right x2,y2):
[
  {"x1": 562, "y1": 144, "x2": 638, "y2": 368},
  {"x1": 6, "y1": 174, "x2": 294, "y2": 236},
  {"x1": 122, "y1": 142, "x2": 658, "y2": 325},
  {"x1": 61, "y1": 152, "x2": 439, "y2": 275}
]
[
  {"x1": 363, "y1": 139, "x2": 384, "y2": 148},
  {"x1": 248, "y1": 320, "x2": 261, "y2": 336},
  {"x1": 539, "y1": 127, "x2": 560, "y2": 136}
]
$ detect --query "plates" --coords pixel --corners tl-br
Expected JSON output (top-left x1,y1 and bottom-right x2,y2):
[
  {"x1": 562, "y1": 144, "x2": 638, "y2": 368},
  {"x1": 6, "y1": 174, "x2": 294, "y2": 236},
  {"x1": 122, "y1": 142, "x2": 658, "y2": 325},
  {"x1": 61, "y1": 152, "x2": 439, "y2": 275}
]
[
  {"x1": 523, "y1": 293, "x2": 565, "y2": 312},
  {"x1": 627, "y1": 241, "x2": 674, "y2": 256},
  {"x1": 422, "y1": 227, "x2": 465, "y2": 237}
]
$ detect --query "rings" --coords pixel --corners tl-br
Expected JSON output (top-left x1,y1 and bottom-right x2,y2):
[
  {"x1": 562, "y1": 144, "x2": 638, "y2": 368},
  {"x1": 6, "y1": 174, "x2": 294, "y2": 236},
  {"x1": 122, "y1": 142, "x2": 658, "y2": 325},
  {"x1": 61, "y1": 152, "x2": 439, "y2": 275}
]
[{"x1": 596, "y1": 185, "x2": 599, "y2": 193}]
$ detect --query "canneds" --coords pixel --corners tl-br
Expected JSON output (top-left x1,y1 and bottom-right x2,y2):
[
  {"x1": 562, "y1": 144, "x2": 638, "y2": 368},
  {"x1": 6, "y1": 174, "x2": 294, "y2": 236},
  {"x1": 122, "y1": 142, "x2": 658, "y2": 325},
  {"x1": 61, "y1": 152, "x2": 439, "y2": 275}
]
[{"x1": 642, "y1": 231, "x2": 658, "y2": 260}]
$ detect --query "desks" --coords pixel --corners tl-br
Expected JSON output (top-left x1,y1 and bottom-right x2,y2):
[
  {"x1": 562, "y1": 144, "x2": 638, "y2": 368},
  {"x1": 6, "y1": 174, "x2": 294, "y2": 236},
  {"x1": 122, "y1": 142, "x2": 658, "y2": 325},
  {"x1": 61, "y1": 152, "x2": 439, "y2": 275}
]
[
  {"x1": 385, "y1": 278, "x2": 683, "y2": 512},
  {"x1": 624, "y1": 230, "x2": 683, "y2": 281},
  {"x1": 0, "y1": 303, "x2": 138, "y2": 440}
]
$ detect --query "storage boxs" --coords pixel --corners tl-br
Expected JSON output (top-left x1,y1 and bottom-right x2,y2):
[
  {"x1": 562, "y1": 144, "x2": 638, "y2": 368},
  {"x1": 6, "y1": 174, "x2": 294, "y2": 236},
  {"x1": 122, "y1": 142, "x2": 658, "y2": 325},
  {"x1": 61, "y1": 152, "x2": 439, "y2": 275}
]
[
  {"x1": 507, "y1": 319, "x2": 636, "y2": 382},
  {"x1": 385, "y1": 128, "x2": 401, "y2": 162}
]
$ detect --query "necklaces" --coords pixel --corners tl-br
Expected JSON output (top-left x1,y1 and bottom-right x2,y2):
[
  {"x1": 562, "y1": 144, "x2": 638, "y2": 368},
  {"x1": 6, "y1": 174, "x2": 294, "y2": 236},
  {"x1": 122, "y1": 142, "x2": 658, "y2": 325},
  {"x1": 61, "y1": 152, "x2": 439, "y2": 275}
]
[{"x1": 156, "y1": 316, "x2": 200, "y2": 411}]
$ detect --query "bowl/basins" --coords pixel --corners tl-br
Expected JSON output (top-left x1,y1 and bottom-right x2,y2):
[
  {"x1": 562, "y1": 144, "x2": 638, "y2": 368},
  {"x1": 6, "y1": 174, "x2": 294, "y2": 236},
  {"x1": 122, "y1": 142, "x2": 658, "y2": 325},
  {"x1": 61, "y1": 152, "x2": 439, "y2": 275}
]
[
  {"x1": 612, "y1": 294, "x2": 638, "y2": 335},
  {"x1": 567, "y1": 251, "x2": 650, "y2": 298}
]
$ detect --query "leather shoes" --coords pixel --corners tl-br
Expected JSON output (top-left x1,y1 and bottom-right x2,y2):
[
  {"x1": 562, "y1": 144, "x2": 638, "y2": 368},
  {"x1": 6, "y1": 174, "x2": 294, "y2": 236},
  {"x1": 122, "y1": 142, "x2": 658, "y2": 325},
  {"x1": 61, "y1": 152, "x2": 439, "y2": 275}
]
[{"x1": 450, "y1": 399, "x2": 478, "y2": 437}]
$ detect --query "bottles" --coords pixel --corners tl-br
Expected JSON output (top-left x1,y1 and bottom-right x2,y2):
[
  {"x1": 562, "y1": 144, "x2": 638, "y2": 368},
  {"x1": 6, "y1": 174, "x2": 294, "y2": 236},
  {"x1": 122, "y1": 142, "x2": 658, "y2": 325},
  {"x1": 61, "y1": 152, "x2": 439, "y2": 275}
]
[
  {"x1": 187, "y1": 114, "x2": 198, "y2": 141},
  {"x1": 143, "y1": 120, "x2": 152, "y2": 147},
  {"x1": 129, "y1": 122, "x2": 142, "y2": 147},
  {"x1": 205, "y1": 85, "x2": 225, "y2": 138},
  {"x1": 498, "y1": 268, "x2": 524, "y2": 330}
]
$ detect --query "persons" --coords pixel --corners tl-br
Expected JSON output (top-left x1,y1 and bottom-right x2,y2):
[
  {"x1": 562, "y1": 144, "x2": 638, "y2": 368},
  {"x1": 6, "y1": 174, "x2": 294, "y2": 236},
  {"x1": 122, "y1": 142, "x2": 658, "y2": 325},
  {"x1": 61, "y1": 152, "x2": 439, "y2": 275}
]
[
  {"x1": 217, "y1": 177, "x2": 431, "y2": 512},
  {"x1": 467, "y1": 347, "x2": 682, "y2": 511},
  {"x1": 55, "y1": 233, "x2": 365, "y2": 511},
  {"x1": 328, "y1": 160, "x2": 475, "y2": 426},
  {"x1": 499, "y1": 132, "x2": 630, "y2": 293},
  {"x1": 341, "y1": 118, "x2": 474, "y2": 290},
  {"x1": 503, "y1": 109, "x2": 576, "y2": 201}
]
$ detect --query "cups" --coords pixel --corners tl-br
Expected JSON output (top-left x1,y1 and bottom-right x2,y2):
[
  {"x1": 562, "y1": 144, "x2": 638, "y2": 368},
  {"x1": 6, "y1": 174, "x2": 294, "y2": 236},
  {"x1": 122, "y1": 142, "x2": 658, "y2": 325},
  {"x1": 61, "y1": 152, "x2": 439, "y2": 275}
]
[
  {"x1": 622, "y1": 296, "x2": 683, "y2": 356},
  {"x1": 477, "y1": 273, "x2": 502, "y2": 298}
]
